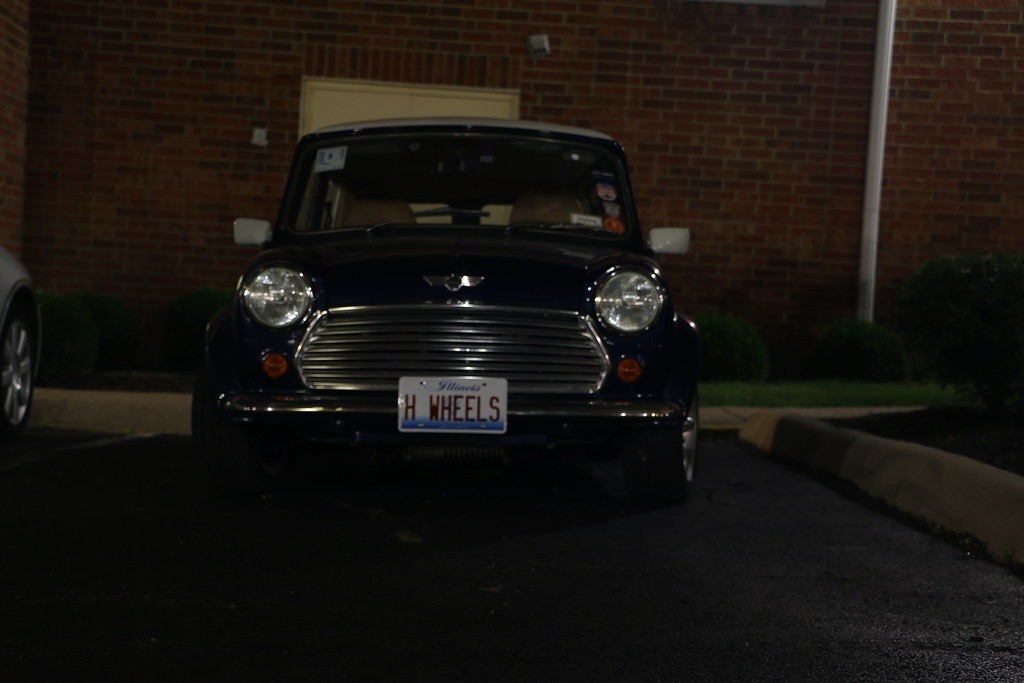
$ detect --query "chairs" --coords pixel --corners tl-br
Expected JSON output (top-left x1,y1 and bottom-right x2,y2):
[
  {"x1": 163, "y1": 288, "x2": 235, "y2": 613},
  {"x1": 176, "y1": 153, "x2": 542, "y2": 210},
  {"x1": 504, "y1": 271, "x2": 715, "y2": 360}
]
[
  {"x1": 340, "y1": 200, "x2": 415, "y2": 225},
  {"x1": 510, "y1": 189, "x2": 576, "y2": 225}
]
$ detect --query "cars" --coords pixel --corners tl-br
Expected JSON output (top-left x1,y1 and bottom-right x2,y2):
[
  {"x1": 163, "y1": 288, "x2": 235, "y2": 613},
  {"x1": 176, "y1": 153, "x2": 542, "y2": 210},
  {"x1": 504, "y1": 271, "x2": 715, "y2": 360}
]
[
  {"x1": 0, "y1": 244, "x2": 43, "y2": 435},
  {"x1": 188, "y1": 116, "x2": 699, "y2": 498}
]
